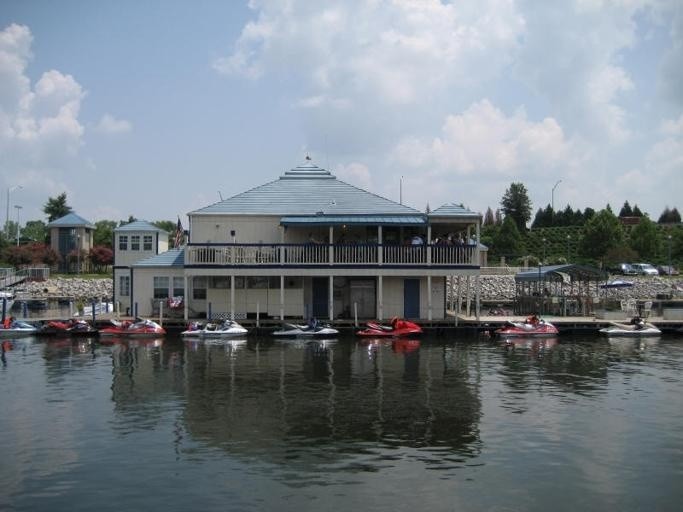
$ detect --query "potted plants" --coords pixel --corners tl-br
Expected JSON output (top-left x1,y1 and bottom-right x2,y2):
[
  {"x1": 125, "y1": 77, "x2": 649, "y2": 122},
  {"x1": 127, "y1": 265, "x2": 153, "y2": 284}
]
[{"x1": 76, "y1": 302, "x2": 85, "y2": 317}]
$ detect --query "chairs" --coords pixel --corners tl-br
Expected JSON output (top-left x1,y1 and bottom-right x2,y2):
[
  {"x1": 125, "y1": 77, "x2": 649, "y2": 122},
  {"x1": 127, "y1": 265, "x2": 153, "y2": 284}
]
[{"x1": 620, "y1": 298, "x2": 653, "y2": 318}]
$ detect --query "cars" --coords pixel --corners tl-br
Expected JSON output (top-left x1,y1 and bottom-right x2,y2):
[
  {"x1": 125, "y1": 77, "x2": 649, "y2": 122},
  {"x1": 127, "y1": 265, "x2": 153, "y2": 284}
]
[{"x1": 599, "y1": 262, "x2": 679, "y2": 288}]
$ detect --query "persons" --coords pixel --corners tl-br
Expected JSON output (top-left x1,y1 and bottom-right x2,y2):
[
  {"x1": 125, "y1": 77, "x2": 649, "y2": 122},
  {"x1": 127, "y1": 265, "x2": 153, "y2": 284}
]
[{"x1": 403, "y1": 230, "x2": 475, "y2": 265}]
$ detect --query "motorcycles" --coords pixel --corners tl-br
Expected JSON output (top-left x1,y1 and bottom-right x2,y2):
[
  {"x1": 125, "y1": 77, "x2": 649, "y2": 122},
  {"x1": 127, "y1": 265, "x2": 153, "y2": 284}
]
[
  {"x1": 494, "y1": 317, "x2": 558, "y2": 336},
  {"x1": 598, "y1": 317, "x2": 662, "y2": 336}
]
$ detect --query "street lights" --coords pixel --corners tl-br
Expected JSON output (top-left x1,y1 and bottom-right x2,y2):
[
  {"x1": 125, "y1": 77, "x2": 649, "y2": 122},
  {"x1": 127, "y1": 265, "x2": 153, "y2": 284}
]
[
  {"x1": 548, "y1": 179, "x2": 563, "y2": 210},
  {"x1": 566, "y1": 235, "x2": 571, "y2": 263},
  {"x1": 668, "y1": 235, "x2": 672, "y2": 275},
  {"x1": 542, "y1": 238, "x2": 546, "y2": 261},
  {"x1": 6, "y1": 183, "x2": 24, "y2": 248},
  {"x1": 77, "y1": 234, "x2": 80, "y2": 275}
]
